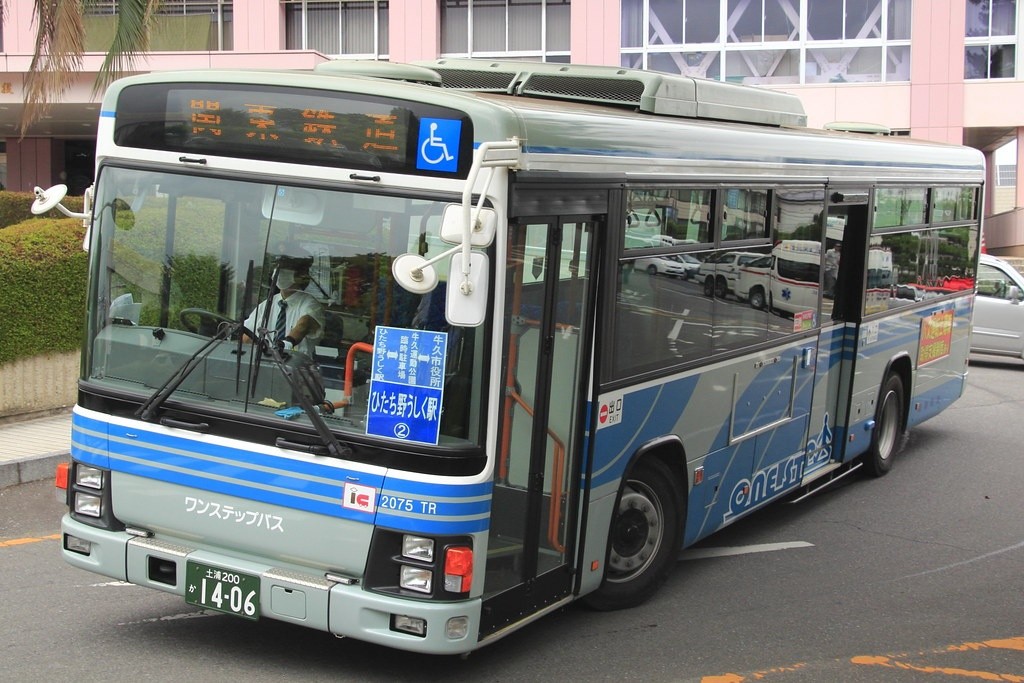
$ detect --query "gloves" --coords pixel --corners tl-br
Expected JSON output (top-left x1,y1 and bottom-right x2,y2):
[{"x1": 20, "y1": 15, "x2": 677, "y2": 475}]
[{"x1": 282, "y1": 340, "x2": 293, "y2": 353}]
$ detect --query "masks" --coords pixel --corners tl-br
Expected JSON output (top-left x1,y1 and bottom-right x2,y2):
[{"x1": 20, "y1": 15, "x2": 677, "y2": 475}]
[{"x1": 271, "y1": 269, "x2": 296, "y2": 289}]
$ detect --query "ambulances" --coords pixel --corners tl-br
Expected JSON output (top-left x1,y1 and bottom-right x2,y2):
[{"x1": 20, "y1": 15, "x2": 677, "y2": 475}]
[{"x1": 726, "y1": 237, "x2": 894, "y2": 326}]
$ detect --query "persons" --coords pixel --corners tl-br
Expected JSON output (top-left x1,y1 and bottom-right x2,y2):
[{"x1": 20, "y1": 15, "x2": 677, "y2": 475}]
[
  {"x1": 823, "y1": 243, "x2": 841, "y2": 299},
  {"x1": 241, "y1": 245, "x2": 326, "y2": 363}
]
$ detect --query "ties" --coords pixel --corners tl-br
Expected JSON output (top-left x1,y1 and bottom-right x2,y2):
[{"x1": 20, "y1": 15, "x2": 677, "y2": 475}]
[{"x1": 272, "y1": 300, "x2": 288, "y2": 348}]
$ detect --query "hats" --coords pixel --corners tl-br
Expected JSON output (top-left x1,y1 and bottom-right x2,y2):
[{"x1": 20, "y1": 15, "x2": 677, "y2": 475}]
[{"x1": 272, "y1": 243, "x2": 314, "y2": 267}]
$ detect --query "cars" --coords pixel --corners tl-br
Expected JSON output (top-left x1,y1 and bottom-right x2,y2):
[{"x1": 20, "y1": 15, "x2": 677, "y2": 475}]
[
  {"x1": 969, "y1": 253, "x2": 1023, "y2": 367},
  {"x1": 623, "y1": 231, "x2": 768, "y2": 301}
]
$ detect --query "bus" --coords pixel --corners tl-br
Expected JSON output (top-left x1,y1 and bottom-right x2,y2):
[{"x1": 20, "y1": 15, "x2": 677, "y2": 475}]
[{"x1": 30, "y1": 53, "x2": 989, "y2": 658}]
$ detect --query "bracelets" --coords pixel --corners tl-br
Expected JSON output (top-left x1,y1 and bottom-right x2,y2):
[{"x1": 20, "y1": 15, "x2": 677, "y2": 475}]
[{"x1": 283, "y1": 335, "x2": 296, "y2": 348}]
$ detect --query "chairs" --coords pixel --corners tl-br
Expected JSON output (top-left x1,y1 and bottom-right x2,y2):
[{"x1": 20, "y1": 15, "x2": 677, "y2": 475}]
[{"x1": 312, "y1": 309, "x2": 344, "y2": 365}]
